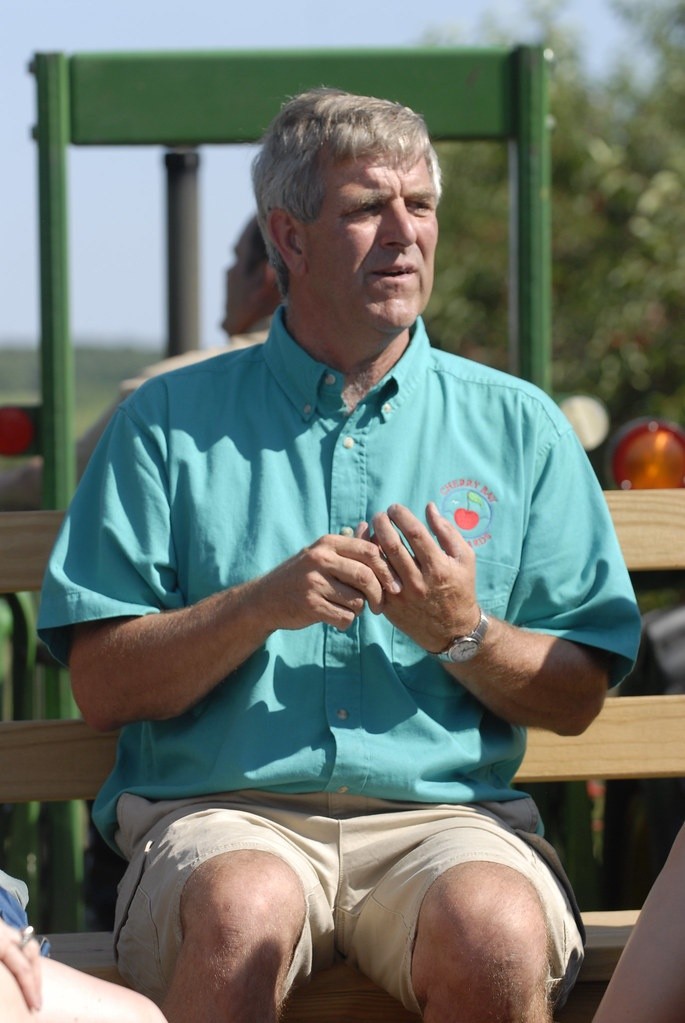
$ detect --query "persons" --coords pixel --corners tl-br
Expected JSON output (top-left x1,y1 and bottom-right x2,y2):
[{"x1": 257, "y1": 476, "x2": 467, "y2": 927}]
[
  {"x1": 591, "y1": 823, "x2": 684, "y2": 1022},
  {"x1": 37, "y1": 85, "x2": 644, "y2": 1023},
  {"x1": 1, "y1": 216, "x2": 283, "y2": 510},
  {"x1": 0, "y1": 869, "x2": 171, "y2": 1022}
]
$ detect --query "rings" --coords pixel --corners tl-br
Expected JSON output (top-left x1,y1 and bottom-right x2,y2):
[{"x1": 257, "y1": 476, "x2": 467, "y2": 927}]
[{"x1": 20, "y1": 926, "x2": 35, "y2": 947}]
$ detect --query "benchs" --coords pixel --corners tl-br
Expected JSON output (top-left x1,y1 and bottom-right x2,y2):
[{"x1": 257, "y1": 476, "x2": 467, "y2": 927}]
[{"x1": 0, "y1": 489, "x2": 685, "y2": 1023}]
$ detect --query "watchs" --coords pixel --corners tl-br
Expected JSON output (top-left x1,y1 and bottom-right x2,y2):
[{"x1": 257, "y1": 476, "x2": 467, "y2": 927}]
[{"x1": 429, "y1": 610, "x2": 489, "y2": 664}]
